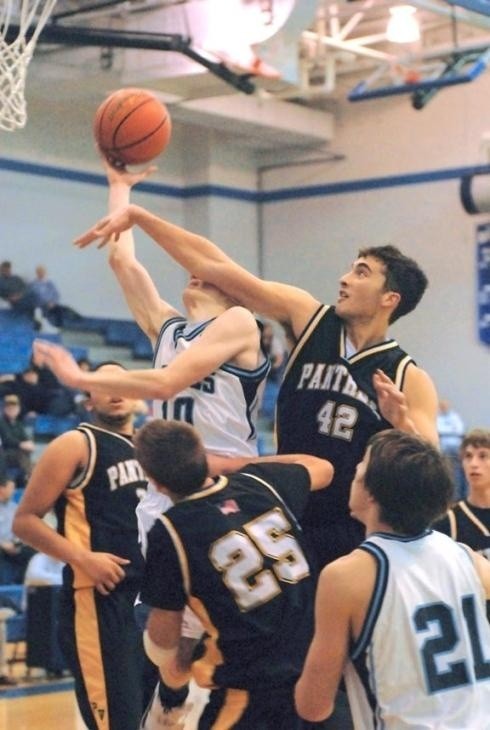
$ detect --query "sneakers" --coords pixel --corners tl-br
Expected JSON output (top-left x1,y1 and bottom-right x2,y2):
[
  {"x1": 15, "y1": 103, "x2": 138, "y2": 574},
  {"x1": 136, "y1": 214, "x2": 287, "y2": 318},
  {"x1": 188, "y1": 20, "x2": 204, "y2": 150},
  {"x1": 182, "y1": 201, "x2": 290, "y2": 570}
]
[{"x1": 140, "y1": 680, "x2": 194, "y2": 730}]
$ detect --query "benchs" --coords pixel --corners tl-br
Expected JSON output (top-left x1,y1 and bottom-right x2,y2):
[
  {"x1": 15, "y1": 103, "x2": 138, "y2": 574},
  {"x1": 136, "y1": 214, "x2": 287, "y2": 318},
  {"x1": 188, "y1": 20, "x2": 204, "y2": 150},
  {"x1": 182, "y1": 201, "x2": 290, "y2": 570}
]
[{"x1": 2, "y1": 308, "x2": 154, "y2": 443}]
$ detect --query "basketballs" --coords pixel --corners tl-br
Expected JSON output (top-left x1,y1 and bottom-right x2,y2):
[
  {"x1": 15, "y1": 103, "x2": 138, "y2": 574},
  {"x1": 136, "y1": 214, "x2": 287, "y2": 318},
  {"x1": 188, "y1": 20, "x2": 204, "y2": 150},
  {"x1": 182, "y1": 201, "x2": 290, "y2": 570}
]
[{"x1": 95, "y1": 88, "x2": 171, "y2": 165}]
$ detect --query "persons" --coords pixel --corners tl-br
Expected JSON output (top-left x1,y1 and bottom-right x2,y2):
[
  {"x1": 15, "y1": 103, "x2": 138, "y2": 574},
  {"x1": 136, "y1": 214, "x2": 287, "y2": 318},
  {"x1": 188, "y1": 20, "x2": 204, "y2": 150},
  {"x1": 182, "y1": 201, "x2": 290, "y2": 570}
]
[{"x1": 1, "y1": 144, "x2": 489, "y2": 729}]
